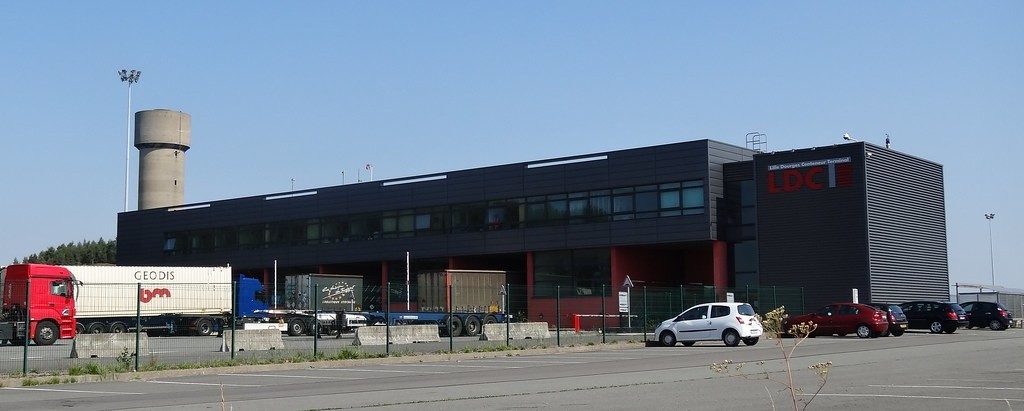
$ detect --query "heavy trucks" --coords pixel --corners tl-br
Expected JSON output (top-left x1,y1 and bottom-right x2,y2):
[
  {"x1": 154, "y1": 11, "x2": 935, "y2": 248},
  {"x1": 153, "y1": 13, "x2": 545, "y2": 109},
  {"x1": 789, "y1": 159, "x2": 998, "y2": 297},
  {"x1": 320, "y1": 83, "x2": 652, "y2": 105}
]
[{"x1": 0, "y1": 263, "x2": 270, "y2": 337}]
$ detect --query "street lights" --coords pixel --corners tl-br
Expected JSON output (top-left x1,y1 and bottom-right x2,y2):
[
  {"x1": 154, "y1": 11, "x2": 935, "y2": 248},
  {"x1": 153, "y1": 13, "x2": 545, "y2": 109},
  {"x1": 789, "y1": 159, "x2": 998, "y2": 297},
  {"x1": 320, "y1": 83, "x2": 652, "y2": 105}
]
[
  {"x1": 117, "y1": 70, "x2": 141, "y2": 211},
  {"x1": 984, "y1": 213, "x2": 996, "y2": 292}
]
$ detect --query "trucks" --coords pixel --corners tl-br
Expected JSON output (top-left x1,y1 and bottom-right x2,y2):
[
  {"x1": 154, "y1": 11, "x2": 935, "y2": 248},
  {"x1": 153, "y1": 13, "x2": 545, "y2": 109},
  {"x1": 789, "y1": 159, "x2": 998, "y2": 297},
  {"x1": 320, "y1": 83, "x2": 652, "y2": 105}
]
[{"x1": 0, "y1": 263, "x2": 83, "y2": 346}]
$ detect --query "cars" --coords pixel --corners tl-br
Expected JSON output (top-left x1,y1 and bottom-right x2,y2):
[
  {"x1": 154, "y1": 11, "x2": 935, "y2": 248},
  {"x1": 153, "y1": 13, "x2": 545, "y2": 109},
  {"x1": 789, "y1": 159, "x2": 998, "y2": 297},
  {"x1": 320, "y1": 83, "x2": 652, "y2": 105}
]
[
  {"x1": 655, "y1": 302, "x2": 764, "y2": 347},
  {"x1": 956, "y1": 301, "x2": 1014, "y2": 331},
  {"x1": 855, "y1": 301, "x2": 909, "y2": 338},
  {"x1": 783, "y1": 302, "x2": 891, "y2": 339},
  {"x1": 897, "y1": 299, "x2": 970, "y2": 334}
]
